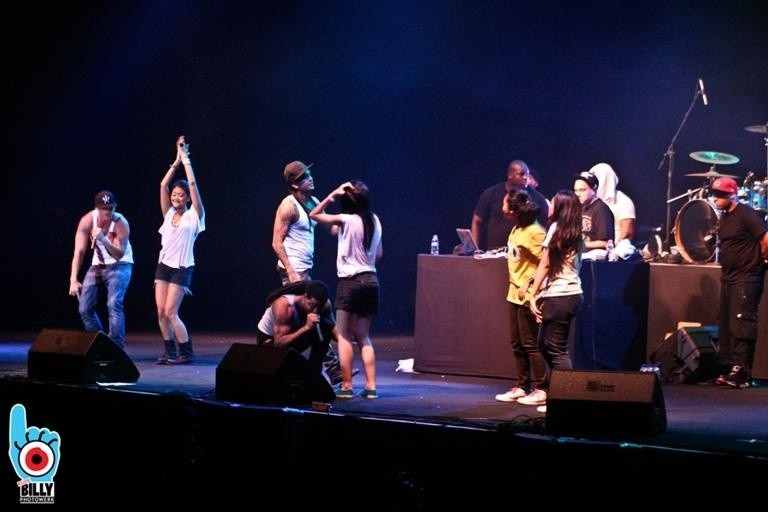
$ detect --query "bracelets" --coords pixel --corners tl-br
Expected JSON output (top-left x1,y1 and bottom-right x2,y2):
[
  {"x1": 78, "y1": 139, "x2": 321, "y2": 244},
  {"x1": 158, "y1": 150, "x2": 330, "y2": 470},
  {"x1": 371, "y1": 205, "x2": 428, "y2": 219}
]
[
  {"x1": 170, "y1": 165, "x2": 178, "y2": 168},
  {"x1": 328, "y1": 194, "x2": 335, "y2": 203},
  {"x1": 182, "y1": 158, "x2": 190, "y2": 165},
  {"x1": 101, "y1": 236, "x2": 109, "y2": 245}
]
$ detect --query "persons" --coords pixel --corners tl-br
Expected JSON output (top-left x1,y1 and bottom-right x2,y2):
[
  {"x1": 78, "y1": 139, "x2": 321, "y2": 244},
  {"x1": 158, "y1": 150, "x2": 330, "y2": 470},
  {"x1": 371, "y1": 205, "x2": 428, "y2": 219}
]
[
  {"x1": 256, "y1": 280, "x2": 342, "y2": 373},
  {"x1": 69, "y1": 192, "x2": 134, "y2": 350},
  {"x1": 272, "y1": 161, "x2": 360, "y2": 385},
  {"x1": 710, "y1": 175, "x2": 768, "y2": 389},
  {"x1": 308, "y1": 179, "x2": 382, "y2": 398},
  {"x1": 737, "y1": 172, "x2": 768, "y2": 214},
  {"x1": 154, "y1": 134, "x2": 207, "y2": 364}
]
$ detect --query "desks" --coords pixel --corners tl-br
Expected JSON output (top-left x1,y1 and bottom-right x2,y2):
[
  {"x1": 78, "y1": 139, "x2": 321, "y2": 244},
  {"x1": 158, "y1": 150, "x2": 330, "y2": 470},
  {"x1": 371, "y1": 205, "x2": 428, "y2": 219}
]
[{"x1": 410, "y1": 252, "x2": 726, "y2": 387}]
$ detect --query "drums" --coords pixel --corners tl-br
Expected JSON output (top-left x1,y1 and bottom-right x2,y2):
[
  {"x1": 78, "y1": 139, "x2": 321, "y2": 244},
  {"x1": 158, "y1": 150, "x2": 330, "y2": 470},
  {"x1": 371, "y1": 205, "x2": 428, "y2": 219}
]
[
  {"x1": 734, "y1": 181, "x2": 768, "y2": 213},
  {"x1": 674, "y1": 200, "x2": 724, "y2": 264}
]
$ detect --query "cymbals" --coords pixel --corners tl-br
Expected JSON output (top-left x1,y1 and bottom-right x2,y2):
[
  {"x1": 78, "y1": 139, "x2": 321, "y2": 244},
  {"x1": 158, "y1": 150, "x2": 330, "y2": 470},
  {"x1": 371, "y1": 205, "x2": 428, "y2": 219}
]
[
  {"x1": 745, "y1": 126, "x2": 768, "y2": 133},
  {"x1": 686, "y1": 172, "x2": 740, "y2": 178},
  {"x1": 687, "y1": 151, "x2": 740, "y2": 165}
]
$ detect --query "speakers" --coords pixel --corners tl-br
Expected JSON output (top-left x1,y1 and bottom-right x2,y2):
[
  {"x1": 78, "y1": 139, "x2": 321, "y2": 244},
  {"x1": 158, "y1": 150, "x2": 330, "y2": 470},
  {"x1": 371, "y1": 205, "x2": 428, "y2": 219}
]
[
  {"x1": 215, "y1": 342, "x2": 336, "y2": 407},
  {"x1": 27, "y1": 328, "x2": 140, "y2": 384},
  {"x1": 546, "y1": 368, "x2": 667, "y2": 437},
  {"x1": 648, "y1": 327, "x2": 722, "y2": 383}
]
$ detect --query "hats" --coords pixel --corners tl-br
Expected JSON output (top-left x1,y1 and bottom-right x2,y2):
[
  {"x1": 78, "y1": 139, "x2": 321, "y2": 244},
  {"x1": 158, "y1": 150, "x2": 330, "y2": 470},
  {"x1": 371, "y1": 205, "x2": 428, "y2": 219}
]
[
  {"x1": 95, "y1": 190, "x2": 118, "y2": 208},
  {"x1": 283, "y1": 160, "x2": 314, "y2": 183},
  {"x1": 705, "y1": 176, "x2": 739, "y2": 195},
  {"x1": 346, "y1": 179, "x2": 371, "y2": 204},
  {"x1": 572, "y1": 171, "x2": 600, "y2": 192}
]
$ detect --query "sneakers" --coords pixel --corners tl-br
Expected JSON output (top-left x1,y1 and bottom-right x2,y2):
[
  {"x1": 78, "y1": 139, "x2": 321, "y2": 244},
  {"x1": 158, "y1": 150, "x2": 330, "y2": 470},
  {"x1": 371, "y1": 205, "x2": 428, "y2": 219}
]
[
  {"x1": 495, "y1": 386, "x2": 548, "y2": 406},
  {"x1": 715, "y1": 365, "x2": 752, "y2": 389},
  {"x1": 334, "y1": 384, "x2": 355, "y2": 399},
  {"x1": 356, "y1": 388, "x2": 378, "y2": 400}
]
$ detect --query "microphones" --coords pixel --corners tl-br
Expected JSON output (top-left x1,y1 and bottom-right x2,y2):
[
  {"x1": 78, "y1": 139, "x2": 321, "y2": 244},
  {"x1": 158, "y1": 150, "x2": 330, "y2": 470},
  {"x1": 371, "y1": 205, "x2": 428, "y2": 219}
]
[
  {"x1": 315, "y1": 322, "x2": 324, "y2": 341},
  {"x1": 90, "y1": 236, "x2": 97, "y2": 250},
  {"x1": 743, "y1": 173, "x2": 754, "y2": 185},
  {"x1": 697, "y1": 78, "x2": 710, "y2": 106}
]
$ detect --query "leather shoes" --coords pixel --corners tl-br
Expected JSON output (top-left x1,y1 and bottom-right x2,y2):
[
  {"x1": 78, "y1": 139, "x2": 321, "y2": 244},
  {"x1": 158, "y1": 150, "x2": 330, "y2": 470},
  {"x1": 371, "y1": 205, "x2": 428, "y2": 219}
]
[{"x1": 325, "y1": 361, "x2": 361, "y2": 386}]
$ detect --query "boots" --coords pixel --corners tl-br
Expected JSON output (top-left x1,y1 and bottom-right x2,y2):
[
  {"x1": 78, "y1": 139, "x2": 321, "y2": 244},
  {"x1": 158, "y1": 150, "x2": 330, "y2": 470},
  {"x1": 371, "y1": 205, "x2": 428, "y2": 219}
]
[{"x1": 157, "y1": 336, "x2": 197, "y2": 366}]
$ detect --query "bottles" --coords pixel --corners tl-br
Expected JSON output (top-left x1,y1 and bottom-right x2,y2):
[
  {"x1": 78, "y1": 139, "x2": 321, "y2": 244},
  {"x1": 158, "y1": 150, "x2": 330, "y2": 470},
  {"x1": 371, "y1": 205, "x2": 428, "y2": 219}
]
[
  {"x1": 606, "y1": 238, "x2": 614, "y2": 251},
  {"x1": 638, "y1": 363, "x2": 664, "y2": 383},
  {"x1": 429, "y1": 235, "x2": 440, "y2": 254}
]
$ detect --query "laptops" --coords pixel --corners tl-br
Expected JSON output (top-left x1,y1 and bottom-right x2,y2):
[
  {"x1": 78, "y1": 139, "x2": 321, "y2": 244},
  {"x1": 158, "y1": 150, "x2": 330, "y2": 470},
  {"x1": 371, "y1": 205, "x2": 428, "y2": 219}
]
[{"x1": 456, "y1": 228, "x2": 484, "y2": 254}]
